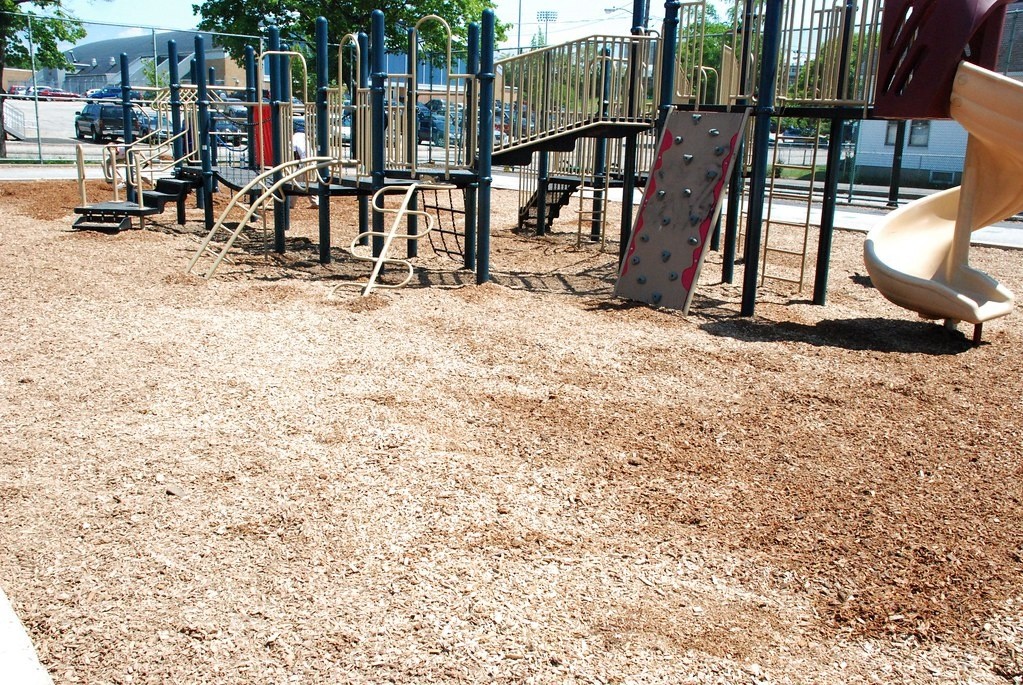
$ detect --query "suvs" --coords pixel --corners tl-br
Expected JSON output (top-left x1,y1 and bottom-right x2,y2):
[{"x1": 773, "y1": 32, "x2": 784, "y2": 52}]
[{"x1": 75, "y1": 100, "x2": 141, "y2": 144}]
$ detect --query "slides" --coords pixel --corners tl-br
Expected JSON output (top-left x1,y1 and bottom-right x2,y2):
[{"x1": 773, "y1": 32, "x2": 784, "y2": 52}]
[{"x1": 863, "y1": 58, "x2": 1023, "y2": 325}]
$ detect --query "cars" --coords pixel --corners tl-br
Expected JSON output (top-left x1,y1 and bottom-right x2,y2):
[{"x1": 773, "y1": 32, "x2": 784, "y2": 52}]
[
  {"x1": 182, "y1": 97, "x2": 247, "y2": 146},
  {"x1": 83, "y1": 82, "x2": 143, "y2": 106},
  {"x1": 8, "y1": 84, "x2": 81, "y2": 102},
  {"x1": 291, "y1": 96, "x2": 565, "y2": 147},
  {"x1": 137, "y1": 113, "x2": 174, "y2": 145}
]
